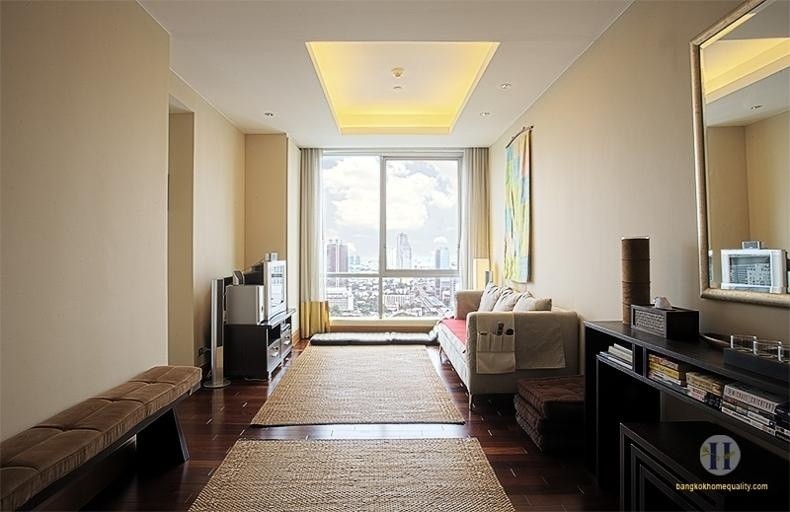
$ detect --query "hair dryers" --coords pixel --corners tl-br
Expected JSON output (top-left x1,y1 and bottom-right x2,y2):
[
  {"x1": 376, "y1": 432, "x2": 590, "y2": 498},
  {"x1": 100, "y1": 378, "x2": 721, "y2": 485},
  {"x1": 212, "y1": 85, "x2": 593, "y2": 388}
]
[{"x1": 631, "y1": 296, "x2": 700, "y2": 341}]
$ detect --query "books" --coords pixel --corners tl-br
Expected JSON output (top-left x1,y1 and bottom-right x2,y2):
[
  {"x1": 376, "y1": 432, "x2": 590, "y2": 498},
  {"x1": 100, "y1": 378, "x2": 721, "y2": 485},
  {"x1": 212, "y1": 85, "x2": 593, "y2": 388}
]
[
  {"x1": 648, "y1": 352, "x2": 790, "y2": 443},
  {"x1": 608, "y1": 346, "x2": 632, "y2": 363},
  {"x1": 599, "y1": 351, "x2": 633, "y2": 372},
  {"x1": 613, "y1": 342, "x2": 633, "y2": 356}
]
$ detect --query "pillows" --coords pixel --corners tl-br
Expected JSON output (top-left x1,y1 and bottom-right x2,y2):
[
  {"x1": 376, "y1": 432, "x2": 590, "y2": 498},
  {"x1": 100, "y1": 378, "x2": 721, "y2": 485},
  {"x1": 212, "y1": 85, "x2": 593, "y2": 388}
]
[
  {"x1": 512, "y1": 291, "x2": 552, "y2": 311},
  {"x1": 491, "y1": 287, "x2": 522, "y2": 312},
  {"x1": 477, "y1": 283, "x2": 504, "y2": 311}
]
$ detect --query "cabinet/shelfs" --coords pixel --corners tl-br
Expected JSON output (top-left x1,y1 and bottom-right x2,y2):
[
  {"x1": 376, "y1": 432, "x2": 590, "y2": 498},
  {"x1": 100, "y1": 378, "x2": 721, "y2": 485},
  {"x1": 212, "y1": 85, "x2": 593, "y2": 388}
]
[
  {"x1": 582, "y1": 321, "x2": 789, "y2": 512},
  {"x1": 224, "y1": 308, "x2": 296, "y2": 381}
]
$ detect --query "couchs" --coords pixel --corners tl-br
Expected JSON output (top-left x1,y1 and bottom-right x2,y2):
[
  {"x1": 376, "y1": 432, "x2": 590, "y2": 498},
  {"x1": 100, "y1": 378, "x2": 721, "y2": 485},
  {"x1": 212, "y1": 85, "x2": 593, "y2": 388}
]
[{"x1": 437, "y1": 289, "x2": 580, "y2": 410}]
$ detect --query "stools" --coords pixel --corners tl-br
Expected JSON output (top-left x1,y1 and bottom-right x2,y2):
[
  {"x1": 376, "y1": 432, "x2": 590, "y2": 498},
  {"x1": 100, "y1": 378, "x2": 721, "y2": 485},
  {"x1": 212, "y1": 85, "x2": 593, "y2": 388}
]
[{"x1": 514, "y1": 374, "x2": 585, "y2": 451}]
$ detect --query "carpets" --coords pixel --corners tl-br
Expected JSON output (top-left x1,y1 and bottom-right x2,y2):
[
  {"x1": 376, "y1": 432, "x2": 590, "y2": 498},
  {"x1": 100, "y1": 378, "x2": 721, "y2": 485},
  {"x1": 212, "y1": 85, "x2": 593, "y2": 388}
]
[
  {"x1": 250, "y1": 333, "x2": 466, "y2": 426},
  {"x1": 188, "y1": 437, "x2": 515, "y2": 512}
]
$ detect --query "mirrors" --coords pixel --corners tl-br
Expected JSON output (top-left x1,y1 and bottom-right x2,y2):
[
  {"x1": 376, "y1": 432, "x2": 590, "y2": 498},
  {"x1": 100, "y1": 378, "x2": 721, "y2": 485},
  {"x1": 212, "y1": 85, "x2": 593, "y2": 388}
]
[{"x1": 688, "y1": 0, "x2": 790, "y2": 307}]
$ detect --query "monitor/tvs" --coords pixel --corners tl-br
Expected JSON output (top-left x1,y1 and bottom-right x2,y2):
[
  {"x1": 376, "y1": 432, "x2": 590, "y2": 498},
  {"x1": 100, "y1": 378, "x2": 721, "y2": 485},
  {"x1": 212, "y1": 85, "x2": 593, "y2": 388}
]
[{"x1": 224, "y1": 260, "x2": 286, "y2": 321}]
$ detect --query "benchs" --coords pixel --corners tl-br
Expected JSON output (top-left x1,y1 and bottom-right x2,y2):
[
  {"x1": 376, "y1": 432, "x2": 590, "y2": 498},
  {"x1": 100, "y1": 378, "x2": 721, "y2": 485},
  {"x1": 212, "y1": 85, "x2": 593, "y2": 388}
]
[{"x1": 0, "y1": 365, "x2": 203, "y2": 512}]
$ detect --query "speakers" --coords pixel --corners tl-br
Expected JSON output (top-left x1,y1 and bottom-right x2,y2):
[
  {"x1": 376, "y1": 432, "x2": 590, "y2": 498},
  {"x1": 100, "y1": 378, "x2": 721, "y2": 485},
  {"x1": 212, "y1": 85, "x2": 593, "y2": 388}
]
[
  {"x1": 233, "y1": 271, "x2": 245, "y2": 286},
  {"x1": 203, "y1": 278, "x2": 232, "y2": 388},
  {"x1": 226, "y1": 284, "x2": 264, "y2": 326}
]
